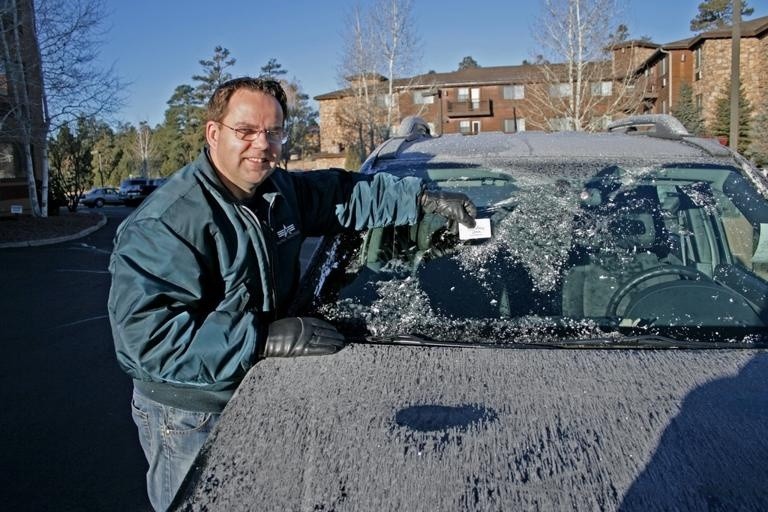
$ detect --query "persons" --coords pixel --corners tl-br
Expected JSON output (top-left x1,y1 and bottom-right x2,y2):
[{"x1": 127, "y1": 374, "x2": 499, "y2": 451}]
[{"x1": 104, "y1": 74, "x2": 480, "y2": 512}]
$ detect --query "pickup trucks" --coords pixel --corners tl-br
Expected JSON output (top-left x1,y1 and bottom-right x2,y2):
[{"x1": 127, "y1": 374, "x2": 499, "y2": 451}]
[{"x1": 126, "y1": 180, "x2": 165, "y2": 200}]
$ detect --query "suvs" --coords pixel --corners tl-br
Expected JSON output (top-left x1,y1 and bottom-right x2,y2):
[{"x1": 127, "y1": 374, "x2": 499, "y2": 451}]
[{"x1": 168, "y1": 111, "x2": 764, "y2": 511}]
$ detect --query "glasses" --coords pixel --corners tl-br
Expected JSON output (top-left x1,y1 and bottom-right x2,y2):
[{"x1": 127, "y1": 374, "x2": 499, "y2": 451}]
[{"x1": 220, "y1": 121, "x2": 290, "y2": 145}]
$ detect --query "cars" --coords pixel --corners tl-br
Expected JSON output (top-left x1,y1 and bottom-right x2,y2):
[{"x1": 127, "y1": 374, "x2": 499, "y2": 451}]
[{"x1": 79, "y1": 187, "x2": 125, "y2": 207}]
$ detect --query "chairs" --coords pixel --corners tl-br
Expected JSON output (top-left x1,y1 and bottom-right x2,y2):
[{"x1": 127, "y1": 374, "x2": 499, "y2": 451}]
[
  {"x1": 391, "y1": 208, "x2": 513, "y2": 320},
  {"x1": 559, "y1": 213, "x2": 682, "y2": 320}
]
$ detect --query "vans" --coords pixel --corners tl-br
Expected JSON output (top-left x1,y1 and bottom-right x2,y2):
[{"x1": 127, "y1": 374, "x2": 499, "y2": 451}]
[{"x1": 118, "y1": 179, "x2": 148, "y2": 200}]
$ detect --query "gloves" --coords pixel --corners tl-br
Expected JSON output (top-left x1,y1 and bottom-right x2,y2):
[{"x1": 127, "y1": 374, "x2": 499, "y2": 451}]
[
  {"x1": 420, "y1": 190, "x2": 477, "y2": 235},
  {"x1": 257, "y1": 316, "x2": 346, "y2": 357}
]
